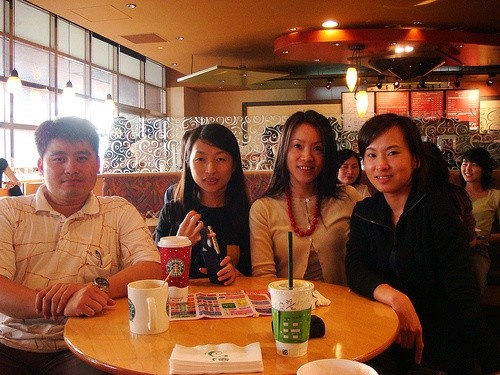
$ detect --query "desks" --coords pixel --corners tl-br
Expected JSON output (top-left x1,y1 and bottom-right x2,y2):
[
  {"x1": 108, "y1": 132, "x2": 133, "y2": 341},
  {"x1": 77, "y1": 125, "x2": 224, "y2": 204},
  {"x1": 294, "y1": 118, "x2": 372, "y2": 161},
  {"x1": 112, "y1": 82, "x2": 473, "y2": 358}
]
[{"x1": 62, "y1": 275, "x2": 400, "y2": 375}]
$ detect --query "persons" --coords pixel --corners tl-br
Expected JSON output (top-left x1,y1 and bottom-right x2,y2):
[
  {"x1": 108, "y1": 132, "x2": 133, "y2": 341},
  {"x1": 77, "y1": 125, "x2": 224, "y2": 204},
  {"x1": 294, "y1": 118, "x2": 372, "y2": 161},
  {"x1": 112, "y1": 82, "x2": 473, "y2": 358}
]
[
  {"x1": 461, "y1": 147, "x2": 500, "y2": 287},
  {"x1": 154, "y1": 123, "x2": 251, "y2": 286},
  {"x1": 0, "y1": 116, "x2": 161, "y2": 375},
  {"x1": 418, "y1": 140, "x2": 477, "y2": 294},
  {"x1": 344, "y1": 114, "x2": 473, "y2": 375},
  {"x1": 249, "y1": 108, "x2": 350, "y2": 286},
  {"x1": 0, "y1": 158, "x2": 23, "y2": 197},
  {"x1": 164, "y1": 166, "x2": 183, "y2": 205},
  {"x1": 335, "y1": 149, "x2": 371, "y2": 199}
]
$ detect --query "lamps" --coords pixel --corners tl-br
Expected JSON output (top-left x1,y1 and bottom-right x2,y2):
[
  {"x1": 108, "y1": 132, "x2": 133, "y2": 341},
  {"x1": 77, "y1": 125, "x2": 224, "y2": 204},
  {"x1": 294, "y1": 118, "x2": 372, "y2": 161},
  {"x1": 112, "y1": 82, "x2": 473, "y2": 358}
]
[
  {"x1": 375, "y1": 75, "x2": 385, "y2": 88},
  {"x1": 454, "y1": 80, "x2": 460, "y2": 88},
  {"x1": 6, "y1": 0, "x2": 22, "y2": 96},
  {"x1": 104, "y1": 94, "x2": 115, "y2": 112},
  {"x1": 488, "y1": 80, "x2": 494, "y2": 86},
  {"x1": 419, "y1": 82, "x2": 426, "y2": 89},
  {"x1": 383, "y1": 57, "x2": 446, "y2": 82},
  {"x1": 63, "y1": 81, "x2": 75, "y2": 99},
  {"x1": 344, "y1": 43, "x2": 369, "y2": 119},
  {"x1": 325, "y1": 78, "x2": 331, "y2": 90},
  {"x1": 394, "y1": 82, "x2": 399, "y2": 88}
]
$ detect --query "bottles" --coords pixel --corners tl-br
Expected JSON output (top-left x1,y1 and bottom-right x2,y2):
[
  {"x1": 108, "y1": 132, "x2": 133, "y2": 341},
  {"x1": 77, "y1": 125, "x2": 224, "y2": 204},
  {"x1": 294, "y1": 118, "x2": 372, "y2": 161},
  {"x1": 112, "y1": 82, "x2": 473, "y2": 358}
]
[{"x1": 200, "y1": 221, "x2": 228, "y2": 284}]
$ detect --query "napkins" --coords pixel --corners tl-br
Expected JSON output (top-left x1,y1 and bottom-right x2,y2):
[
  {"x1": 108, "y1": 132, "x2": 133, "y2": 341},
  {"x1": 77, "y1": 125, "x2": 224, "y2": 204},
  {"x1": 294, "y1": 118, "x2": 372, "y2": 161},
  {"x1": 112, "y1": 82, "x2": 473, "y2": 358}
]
[{"x1": 168, "y1": 341, "x2": 264, "y2": 375}]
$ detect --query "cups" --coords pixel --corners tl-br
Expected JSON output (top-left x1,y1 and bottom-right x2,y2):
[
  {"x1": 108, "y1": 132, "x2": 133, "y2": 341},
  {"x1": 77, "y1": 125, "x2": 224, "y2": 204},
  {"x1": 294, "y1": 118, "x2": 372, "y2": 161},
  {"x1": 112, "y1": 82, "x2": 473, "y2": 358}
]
[
  {"x1": 268, "y1": 279, "x2": 314, "y2": 357},
  {"x1": 127, "y1": 279, "x2": 170, "y2": 335},
  {"x1": 157, "y1": 235, "x2": 192, "y2": 297},
  {"x1": 296, "y1": 358, "x2": 378, "y2": 375}
]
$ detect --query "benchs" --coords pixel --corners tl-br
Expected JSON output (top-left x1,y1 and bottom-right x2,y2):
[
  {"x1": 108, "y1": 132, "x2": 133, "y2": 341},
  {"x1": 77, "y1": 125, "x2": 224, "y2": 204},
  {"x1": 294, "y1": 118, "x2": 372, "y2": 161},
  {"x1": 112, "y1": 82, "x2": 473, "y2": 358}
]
[{"x1": 20, "y1": 169, "x2": 278, "y2": 222}]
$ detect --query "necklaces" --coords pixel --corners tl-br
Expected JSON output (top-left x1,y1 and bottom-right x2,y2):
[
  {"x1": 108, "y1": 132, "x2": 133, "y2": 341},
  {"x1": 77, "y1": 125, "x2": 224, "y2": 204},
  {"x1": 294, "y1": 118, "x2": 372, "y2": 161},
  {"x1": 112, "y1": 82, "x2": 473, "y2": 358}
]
[{"x1": 286, "y1": 190, "x2": 320, "y2": 237}]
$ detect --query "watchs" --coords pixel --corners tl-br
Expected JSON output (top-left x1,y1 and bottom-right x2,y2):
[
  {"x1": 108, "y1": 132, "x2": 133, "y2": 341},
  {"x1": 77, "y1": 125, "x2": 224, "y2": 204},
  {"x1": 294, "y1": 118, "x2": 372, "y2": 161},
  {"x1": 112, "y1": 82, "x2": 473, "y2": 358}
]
[{"x1": 94, "y1": 276, "x2": 111, "y2": 294}]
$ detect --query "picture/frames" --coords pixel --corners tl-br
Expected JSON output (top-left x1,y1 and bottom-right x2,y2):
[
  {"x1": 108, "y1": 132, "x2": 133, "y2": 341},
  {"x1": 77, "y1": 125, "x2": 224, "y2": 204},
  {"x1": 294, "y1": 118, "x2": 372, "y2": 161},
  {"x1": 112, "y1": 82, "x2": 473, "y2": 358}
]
[{"x1": 437, "y1": 135, "x2": 456, "y2": 152}]
